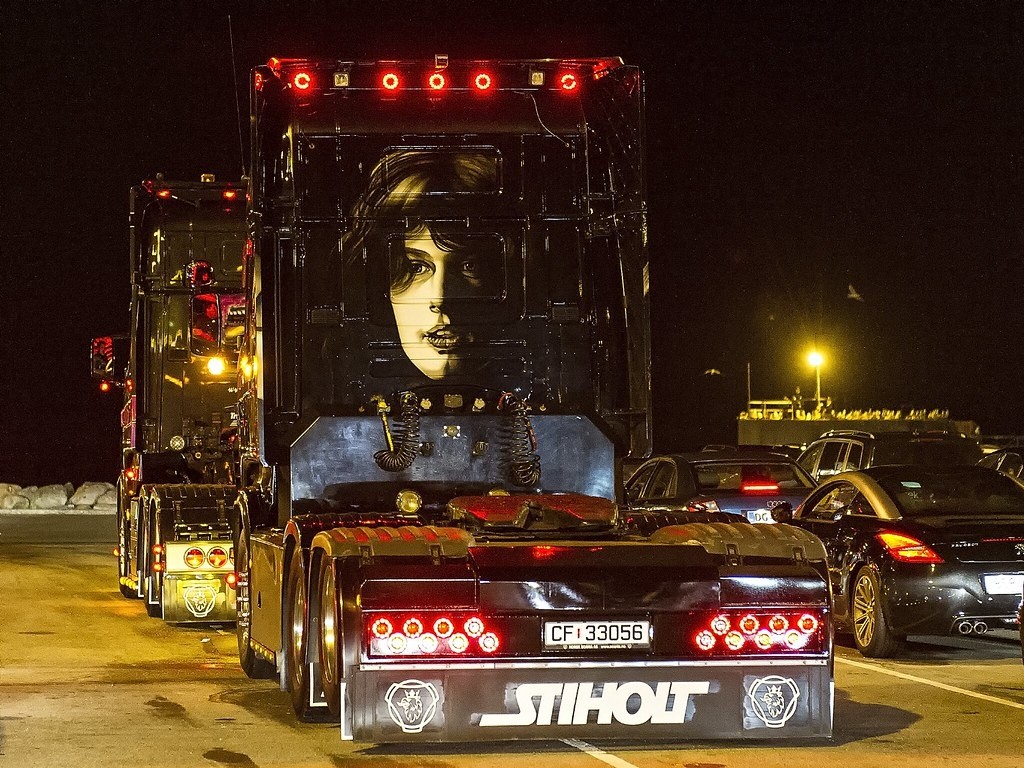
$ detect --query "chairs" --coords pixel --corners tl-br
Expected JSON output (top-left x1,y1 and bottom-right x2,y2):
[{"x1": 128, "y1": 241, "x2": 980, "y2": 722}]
[{"x1": 698, "y1": 473, "x2": 720, "y2": 490}]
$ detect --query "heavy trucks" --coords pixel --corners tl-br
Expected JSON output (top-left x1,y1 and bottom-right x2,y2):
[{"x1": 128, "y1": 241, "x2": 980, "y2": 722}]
[
  {"x1": 92, "y1": 172, "x2": 250, "y2": 624},
  {"x1": 187, "y1": 55, "x2": 835, "y2": 744}
]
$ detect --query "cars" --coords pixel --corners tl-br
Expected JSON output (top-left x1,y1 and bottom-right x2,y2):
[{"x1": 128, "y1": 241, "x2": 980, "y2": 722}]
[
  {"x1": 626, "y1": 449, "x2": 819, "y2": 525},
  {"x1": 976, "y1": 448, "x2": 1024, "y2": 490},
  {"x1": 770, "y1": 463, "x2": 1024, "y2": 657}
]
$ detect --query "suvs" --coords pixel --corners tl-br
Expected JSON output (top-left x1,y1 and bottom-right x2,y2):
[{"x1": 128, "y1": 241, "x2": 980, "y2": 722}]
[{"x1": 797, "y1": 428, "x2": 978, "y2": 485}]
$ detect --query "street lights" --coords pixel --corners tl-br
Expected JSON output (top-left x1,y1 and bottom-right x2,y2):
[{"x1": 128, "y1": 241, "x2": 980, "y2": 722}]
[{"x1": 807, "y1": 348, "x2": 823, "y2": 412}]
